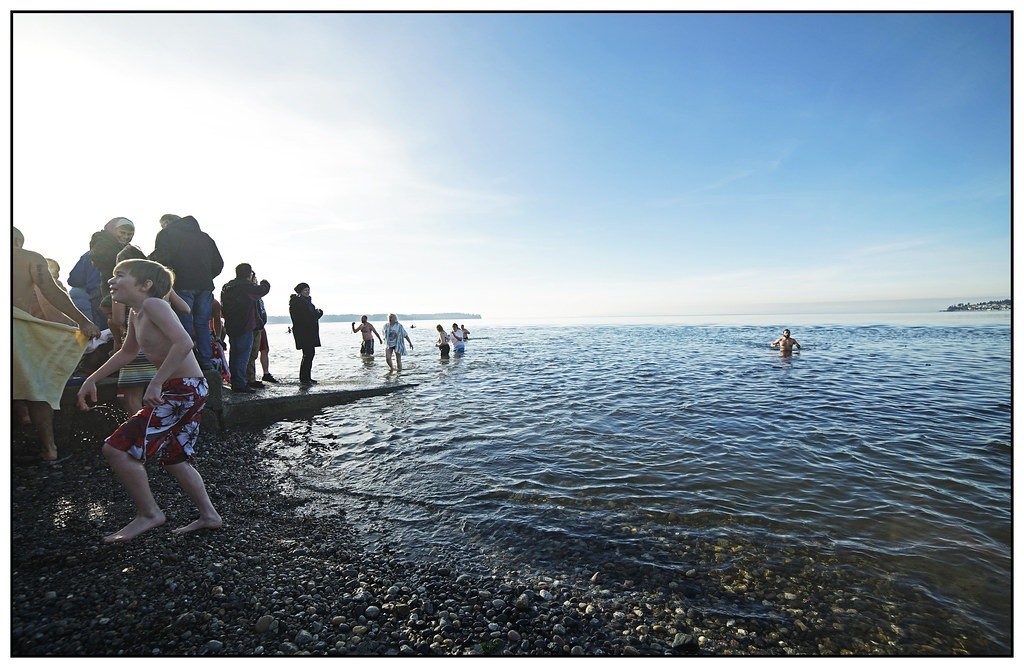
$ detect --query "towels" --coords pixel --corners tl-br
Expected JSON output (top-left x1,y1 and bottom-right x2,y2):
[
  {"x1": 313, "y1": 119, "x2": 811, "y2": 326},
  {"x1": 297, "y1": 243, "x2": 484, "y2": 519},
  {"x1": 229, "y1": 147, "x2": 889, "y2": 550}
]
[{"x1": 12, "y1": 305, "x2": 93, "y2": 411}]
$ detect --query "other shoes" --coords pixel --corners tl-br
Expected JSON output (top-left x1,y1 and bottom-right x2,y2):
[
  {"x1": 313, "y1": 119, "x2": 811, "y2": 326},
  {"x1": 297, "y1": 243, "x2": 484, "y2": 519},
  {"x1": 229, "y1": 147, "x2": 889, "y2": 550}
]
[
  {"x1": 251, "y1": 380, "x2": 265, "y2": 387},
  {"x1": 245, "y1": 387, "x2": 256, "y2": 393},
  {"x1": 301, "y1": 377, "x2": 317, "y2": 385},
  {"x1": 262, "y1": 374, "x2": 278, "y2": 384}
]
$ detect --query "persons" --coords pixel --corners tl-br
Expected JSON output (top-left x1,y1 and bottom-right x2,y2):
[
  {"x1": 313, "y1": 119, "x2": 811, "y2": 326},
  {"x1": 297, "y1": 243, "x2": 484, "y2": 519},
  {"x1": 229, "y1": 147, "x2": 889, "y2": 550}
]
[
  {"x1": 289, "y1": 283, "x2": 323, "y2": 383},
  {"x1": 448, "y1": 323, "x2": 465, "y2": 355},
  {"x1": 435, "y1": 325, "x2": 450, "y2": 357},
  {"x1": 461, "y1": 325, "x2": 470, "y2": 339},
  {"x1": 352, "y1": 315, "x2": 382, "y2": 354},
  {"x1": 383, "y1": 314, "x2": 414, "y2": 371},
  {"x1": 76, "y1": 258, "x2": 222, "y2": 542},
  {"x1": 771, "y1": 329, "x2": 800, "y2": 352},
  {"x1": 14, "y1": 215, "x2": 279, "y2": 460}
]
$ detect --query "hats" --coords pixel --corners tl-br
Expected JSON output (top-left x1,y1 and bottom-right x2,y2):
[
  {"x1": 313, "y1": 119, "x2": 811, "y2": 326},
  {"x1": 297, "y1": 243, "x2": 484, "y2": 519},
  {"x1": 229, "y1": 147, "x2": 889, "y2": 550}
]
[
  {"x1": 105, "y1": 217, "x2": 135, "y2": 231},
  {"x1": 294, "y1": 283, "x2": 309, "y2": 293}
]
